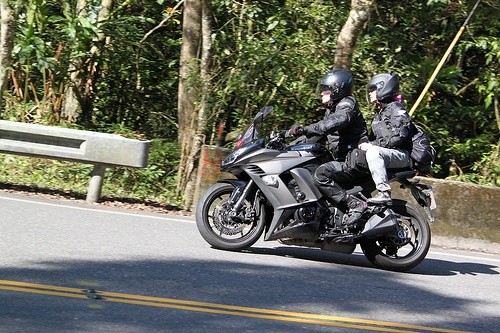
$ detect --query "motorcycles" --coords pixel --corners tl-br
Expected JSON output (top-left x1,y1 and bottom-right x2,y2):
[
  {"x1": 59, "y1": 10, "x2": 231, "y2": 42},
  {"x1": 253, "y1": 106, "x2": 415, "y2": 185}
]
[{"x1": 194, "y1": 106, "x2": 437, "y2": 273}]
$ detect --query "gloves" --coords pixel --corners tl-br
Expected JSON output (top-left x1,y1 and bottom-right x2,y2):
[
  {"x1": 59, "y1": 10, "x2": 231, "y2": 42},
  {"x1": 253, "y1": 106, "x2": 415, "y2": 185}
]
[{"x1": 288, "y1": 125, "x2": 305, "y2": 138}]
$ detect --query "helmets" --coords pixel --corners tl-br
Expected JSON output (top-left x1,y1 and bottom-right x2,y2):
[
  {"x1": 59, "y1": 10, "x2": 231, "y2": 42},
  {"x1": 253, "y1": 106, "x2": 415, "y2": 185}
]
[
  {"x1": 366, "y1": 74, "x2": 400, "y2": 99},
  {"x1": 316, "y1": 71, "x2": 353, "y2": 108}
]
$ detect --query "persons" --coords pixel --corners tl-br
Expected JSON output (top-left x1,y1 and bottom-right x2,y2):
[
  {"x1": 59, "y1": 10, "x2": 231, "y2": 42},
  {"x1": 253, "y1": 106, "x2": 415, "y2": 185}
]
[
  {"x1": 362, "y1": 73, "x2": 417, "y2": 205},
  {"x1": 290, "y1": 69, "x2": 371, "y2": 225}
]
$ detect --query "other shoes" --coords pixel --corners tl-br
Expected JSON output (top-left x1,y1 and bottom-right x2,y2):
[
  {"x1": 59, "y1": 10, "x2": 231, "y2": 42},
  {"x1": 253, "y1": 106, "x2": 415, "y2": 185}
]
[
  {"x1": 343, "y1": 195, "x2": 368, "y2": 226},
  {"x1": 367, "y1": 191, "x2": 392, "y2": 206}
]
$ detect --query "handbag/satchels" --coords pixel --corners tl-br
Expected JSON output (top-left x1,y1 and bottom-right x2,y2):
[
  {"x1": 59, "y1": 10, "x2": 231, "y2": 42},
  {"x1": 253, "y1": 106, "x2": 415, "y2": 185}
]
[{"x1": 407, "y1": 122, "x2": 435, "y2": 167}]
